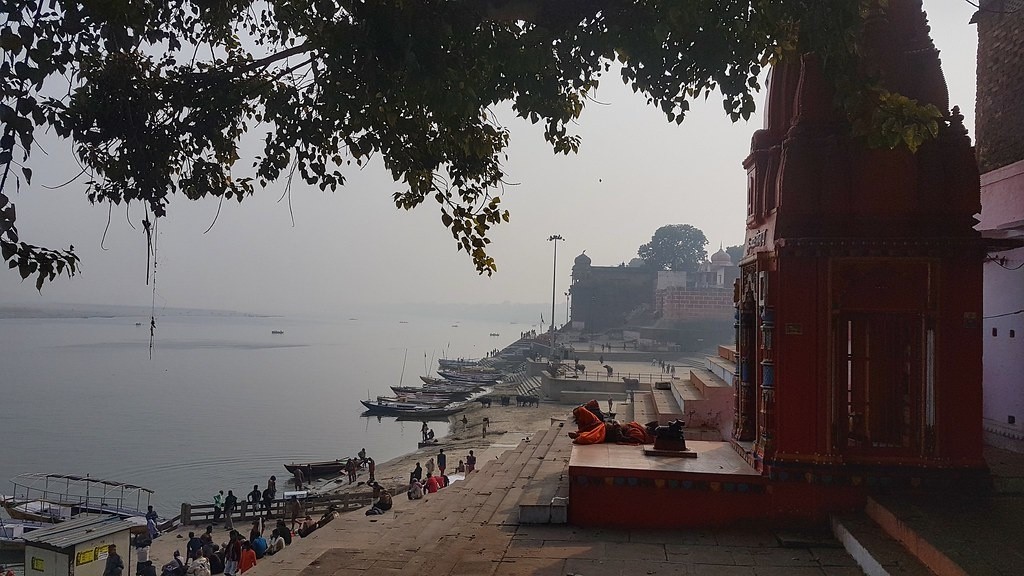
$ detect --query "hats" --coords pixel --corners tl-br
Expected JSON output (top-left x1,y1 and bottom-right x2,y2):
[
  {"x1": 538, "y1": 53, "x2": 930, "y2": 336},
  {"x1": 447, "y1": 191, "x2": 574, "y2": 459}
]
[{"x1": 411, "y1": 478, "x2": 418, "y2": 482}]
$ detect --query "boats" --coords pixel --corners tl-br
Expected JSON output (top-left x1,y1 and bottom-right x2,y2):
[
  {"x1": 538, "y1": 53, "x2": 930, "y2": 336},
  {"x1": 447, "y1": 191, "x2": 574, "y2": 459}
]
[
  {"x1": 283, "y1": 460, "x2": 345, "y2": 476},
  {"x1": 1, "y1": 473, "x2": 171, "y2": 531},
  {"x1": 360, "y1": 345, "x2": 504, "y2": 418}
]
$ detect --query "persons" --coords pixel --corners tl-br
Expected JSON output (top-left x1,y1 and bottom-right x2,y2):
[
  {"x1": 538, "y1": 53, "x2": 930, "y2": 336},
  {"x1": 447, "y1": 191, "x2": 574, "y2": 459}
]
[
  {"x1": 407, "y1": 448, "x2": 477, "y2": 500},
  {"x1": 608, "y1": 394, "x2": 613, "y2": 411},
  {"x1": 460, "y1": 414, "x2": 468, "y2": 431},
  {"x1": 135, "y1": 518, "x2": 292, "y2": 576},
  {"x1": 481, "y1": 417, "x2": 490, "y2": 439},
  {"x1": 291, "y1": 495, "x2": 302, "y2": 528},
  {"x1": 320, "y1": 504, "x2": 340, "y2": 521},
  {"x1": 528, "y1": 350, "x2": 542, "y2": 362},
  {"x1": 487, "y1": 347, "x2": 499, "y2": 358},
  {"x1": 630, "y1": 389, "x2": 634, "y2": 403},
  {"x1": 102, "y1": 544, "x2": 124, "y2": 576},
  {"x1": 298, "y1": 515, "x2": 316, "y2": 533},
  {"x1": 146, "y1": 506, "x2": 160, "y2": 539},
  {"x1": 294, "y1": 464, "x2": 312, "y2": 491},
  {"x1": 213, "y1": 475, "x2": 277, "y2": 521},
  {"x1": 651, "y1": 358, "x2": 675, "y2": 376},
  {"x1": 420, "y1": 421, "x2": 434, "y2": 443},
  {"x1": 345, "y1": 448, "x2": 375, "y2": 485},
  {"x1": 561, "y1": 342, "x2": 626, "y2": 377},
  {"x1": 521, "y1": 329, "x2": 537, "y2": 340},
  {"x1": 568, "y1": 399, "x2": 655, "y2": 444},
  {"x1": 367, "y1": 480, "x2": 393, "y2": 511}
]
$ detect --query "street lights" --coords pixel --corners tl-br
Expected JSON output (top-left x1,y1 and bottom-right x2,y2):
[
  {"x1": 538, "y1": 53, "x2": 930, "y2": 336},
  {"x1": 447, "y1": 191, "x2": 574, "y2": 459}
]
[
  {"x1": 547, "y1": 234, "x2": 565, "y2": 361},
  {"x1": 564, "y1": 292, "x2": 570, "y2": 323}
]
